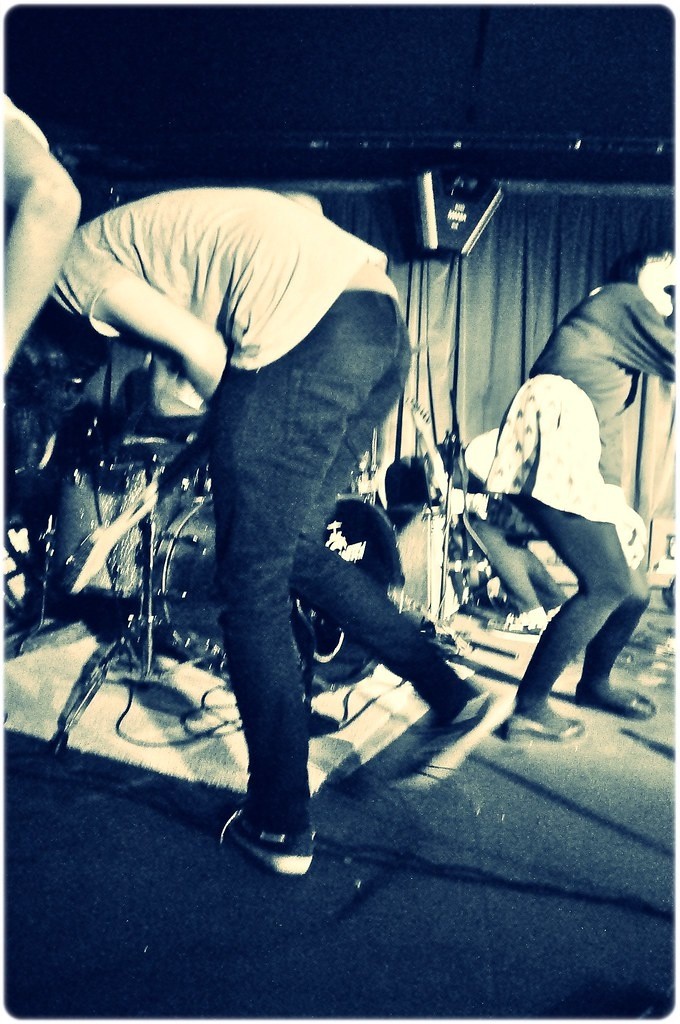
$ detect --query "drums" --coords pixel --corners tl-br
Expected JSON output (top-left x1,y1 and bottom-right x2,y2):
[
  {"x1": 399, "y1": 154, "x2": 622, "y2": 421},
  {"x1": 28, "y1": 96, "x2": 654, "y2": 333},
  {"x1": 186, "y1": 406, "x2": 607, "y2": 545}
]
[
  {"x1": 53, "y1": 435, "x2": 212, "y2": 601},
  {"x1": 148, "y1": 354, "x2": 207, "y2": 420},
  {"x1": 152, "y1": 491, "x2": 406, "y2": 693}
]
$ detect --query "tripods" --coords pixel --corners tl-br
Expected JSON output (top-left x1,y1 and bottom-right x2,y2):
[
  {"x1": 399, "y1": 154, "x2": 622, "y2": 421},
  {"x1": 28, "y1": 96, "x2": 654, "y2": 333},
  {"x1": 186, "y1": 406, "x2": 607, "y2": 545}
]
[{"x1": 44, "y1": 458, "x2": 227, "y2": 761}]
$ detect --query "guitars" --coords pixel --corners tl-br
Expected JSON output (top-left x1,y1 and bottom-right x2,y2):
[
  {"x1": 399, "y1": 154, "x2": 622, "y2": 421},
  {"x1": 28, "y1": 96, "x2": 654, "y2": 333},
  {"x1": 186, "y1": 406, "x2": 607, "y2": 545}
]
[{"x1": 407, "y1": 397, "x2": 511, "y2": 612}]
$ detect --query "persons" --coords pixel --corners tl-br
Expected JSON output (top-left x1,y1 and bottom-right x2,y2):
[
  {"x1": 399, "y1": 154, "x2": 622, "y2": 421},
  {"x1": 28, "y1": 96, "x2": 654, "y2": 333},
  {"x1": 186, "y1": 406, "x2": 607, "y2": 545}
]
[
  {"x1": 2, "y1": 91, "x2": 81, "y2": 380},
  {"x1": 485, "y1": 248, "x2": 675, "y2": 740},
  {"x1": 49, "y1": 185, "x2": 510, "y2": 878},
  {"x1": 426, "y1": 426, "x2": 568, "y2": 634}
]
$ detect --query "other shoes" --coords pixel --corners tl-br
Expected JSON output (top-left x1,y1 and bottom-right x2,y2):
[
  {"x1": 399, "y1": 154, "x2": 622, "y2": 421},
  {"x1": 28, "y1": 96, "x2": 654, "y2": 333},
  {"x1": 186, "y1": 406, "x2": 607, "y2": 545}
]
[
  {"x1": 218, "y1": 802, "x2": 317, "y2": 876},
  {"x1": 509, "y1": 714, "x2": 586, "y2": 741},
  {"x1": 421, "y1": 679, "x2": 494, "y2": 741},
  {"x1": 576, "y1": 687, "x2": 658, "y2": 720}
]
